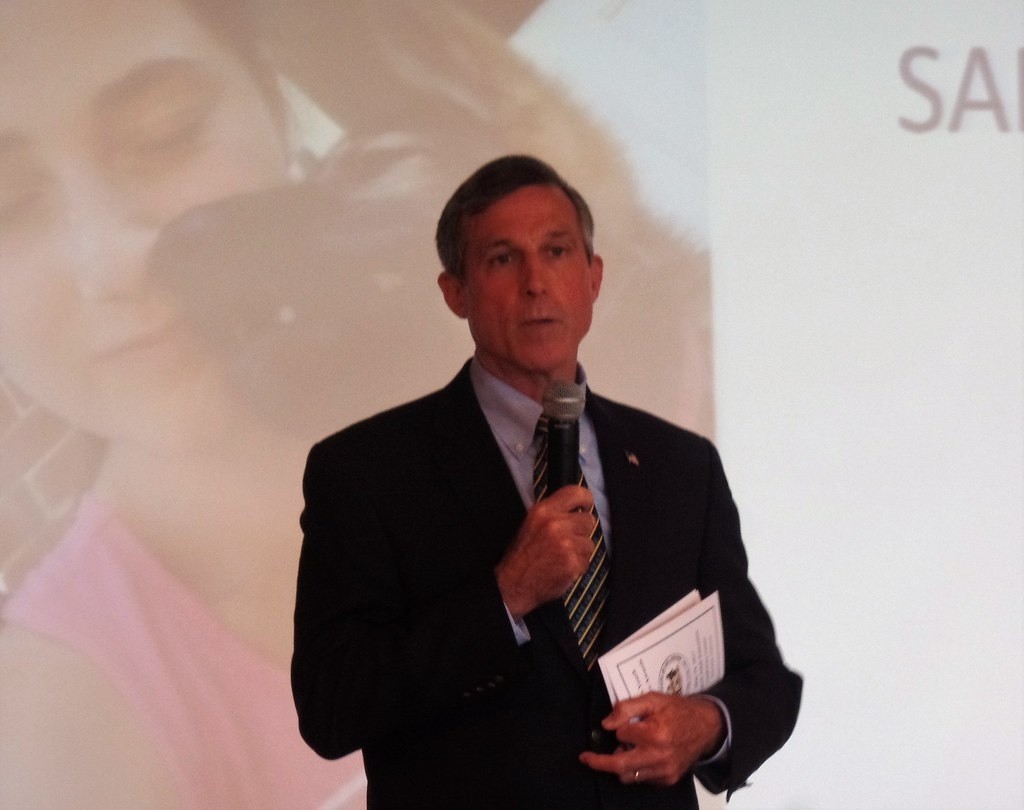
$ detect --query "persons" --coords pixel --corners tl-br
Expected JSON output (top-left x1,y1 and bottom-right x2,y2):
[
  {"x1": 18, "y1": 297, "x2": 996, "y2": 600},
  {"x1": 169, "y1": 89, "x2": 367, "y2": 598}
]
[{"x1": 290, "y1": 155, "x2": 802, "y2": 810}]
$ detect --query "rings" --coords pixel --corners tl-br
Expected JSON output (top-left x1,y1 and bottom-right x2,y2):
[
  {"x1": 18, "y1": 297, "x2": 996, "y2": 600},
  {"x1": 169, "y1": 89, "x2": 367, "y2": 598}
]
[{"x1": 634, "y1": 769, "x2": 640, "y2": 784}]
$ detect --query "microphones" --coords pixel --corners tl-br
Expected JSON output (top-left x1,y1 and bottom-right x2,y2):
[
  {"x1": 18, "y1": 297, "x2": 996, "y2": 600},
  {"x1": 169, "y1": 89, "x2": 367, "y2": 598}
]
[{"x1": 544, "y1": 379, "x2": 587, "y2": 496}]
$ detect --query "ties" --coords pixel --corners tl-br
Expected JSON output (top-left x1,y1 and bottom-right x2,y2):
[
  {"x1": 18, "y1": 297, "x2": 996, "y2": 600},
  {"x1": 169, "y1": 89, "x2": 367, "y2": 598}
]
[{"x1": 530, "y1": 414, "x2": 610, "y2": 676}]
